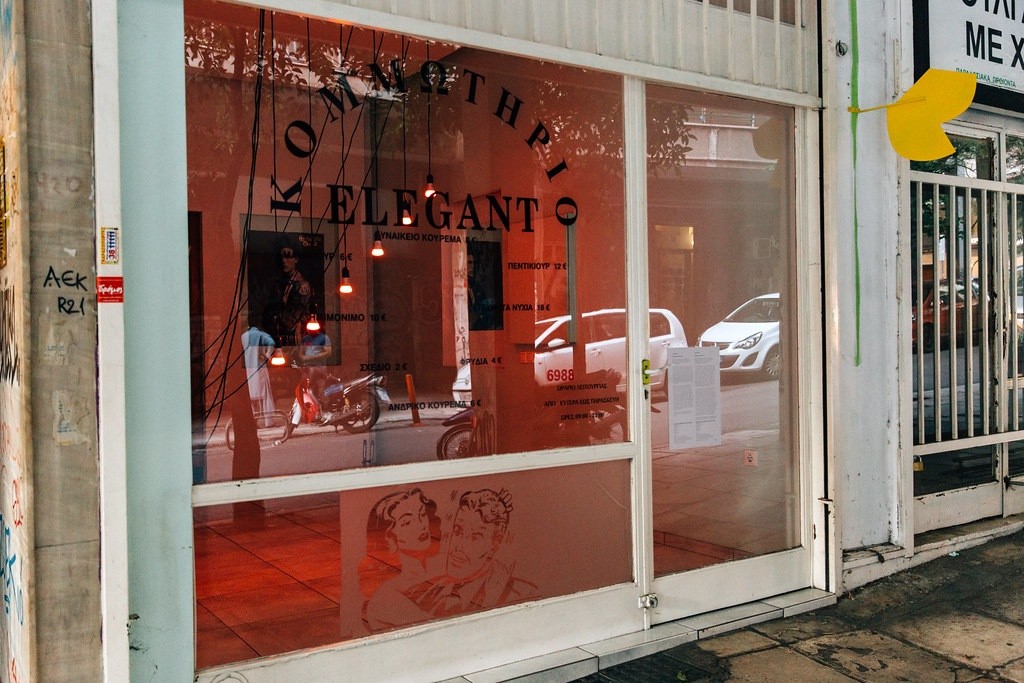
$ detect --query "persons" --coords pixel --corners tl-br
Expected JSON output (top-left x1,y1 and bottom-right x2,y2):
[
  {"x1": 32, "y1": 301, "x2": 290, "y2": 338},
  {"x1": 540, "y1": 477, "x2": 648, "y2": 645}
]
[
  {"x1": 297, "y1": 330, "x2": 332, "y2": 371},
  {"x1": 468, "y1": 252, "x2": 490, "y2": 331},
  {"x1": 261, "y1": 244, "x2": 311, "y2": 346},
  {"x1": 241, "y1": 315, "x2": 285, "y2": 429}
]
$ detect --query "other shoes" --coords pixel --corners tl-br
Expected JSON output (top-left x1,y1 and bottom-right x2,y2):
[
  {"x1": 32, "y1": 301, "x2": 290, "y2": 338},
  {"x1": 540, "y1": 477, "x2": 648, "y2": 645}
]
[{"x1": 267, "y1": 423, "x2": 281, "y2": 428}]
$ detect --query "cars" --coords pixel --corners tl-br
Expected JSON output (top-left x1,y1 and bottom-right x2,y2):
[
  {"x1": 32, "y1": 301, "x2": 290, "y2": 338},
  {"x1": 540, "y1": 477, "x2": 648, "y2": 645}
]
[
  {"x1": 914, "y1": 278, "x2": 1000, "y2": 347},
  {"x1": 1009, "y1": 265, "x2": 1024, "y2": 374},
  {"x1": 697, "y1": 292, "x2": 780, "y2": 380},
  {"x1": 453, "y1": 307, "x2": 691, "y2": 406}
]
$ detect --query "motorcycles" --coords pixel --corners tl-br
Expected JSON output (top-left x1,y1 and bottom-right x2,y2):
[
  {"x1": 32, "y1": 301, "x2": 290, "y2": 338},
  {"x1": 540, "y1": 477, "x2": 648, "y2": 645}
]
[
  {"x1": 436, "y1": 367, "x2": 628, "y2": 461},
  {"x1": 284, "y1": 355, "x2": 391, "y2": 438}
]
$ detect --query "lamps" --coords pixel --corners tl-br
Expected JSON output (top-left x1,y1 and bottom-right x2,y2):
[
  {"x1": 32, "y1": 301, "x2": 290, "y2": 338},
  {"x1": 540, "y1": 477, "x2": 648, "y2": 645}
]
[
  {"x1": 340, "y1": 24, "x2": 353, "y2": 293},
  {"x1": 424, "y1": 41, "x2": 436, "y2": 198},
  {"x1": 271, "y1": 11, "x2": 285, "y2": 365},
  {"x1": 307, "y1": 16, "x2": 321, "y2": 331},
  {"x1": 402, "y1": 36, "x2": 412, "y2": 225},
  {"x1": 372, "y1": 29, "x2": 384, "y2": 257}
]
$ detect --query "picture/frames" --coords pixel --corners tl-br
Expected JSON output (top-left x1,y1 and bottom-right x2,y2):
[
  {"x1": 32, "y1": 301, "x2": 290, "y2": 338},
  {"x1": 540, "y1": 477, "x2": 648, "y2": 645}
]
[{"x1": 239, "y1": 213, "x2": 341, "y2": 368}]
